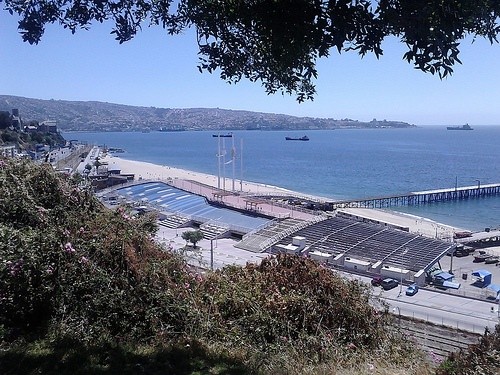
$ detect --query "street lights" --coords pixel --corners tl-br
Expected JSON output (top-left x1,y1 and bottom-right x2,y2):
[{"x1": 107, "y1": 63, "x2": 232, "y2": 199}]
[{"x1": 210, "y1": 235, "x2": 219, "y2": 272}]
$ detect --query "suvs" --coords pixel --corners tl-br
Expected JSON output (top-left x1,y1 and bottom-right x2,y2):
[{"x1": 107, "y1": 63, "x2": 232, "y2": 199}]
[{"x1": 383, "y1": 278, "x2": 398, "y2": 289}]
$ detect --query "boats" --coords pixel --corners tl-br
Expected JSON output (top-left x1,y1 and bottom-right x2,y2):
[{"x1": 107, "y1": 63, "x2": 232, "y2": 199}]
[
  {"x1": 156, "y1": 123, "x2": 187, "y2": 131},
  {"x1": 484, "y1": 256, "x2": 500, "y2": 264},
  {"x1": 285, "y1": 136, "x2": 309, "y2": 141},
  {"x1": 213, "y1": 132, "x2": 233, "y2": 137},
  {"x1": 447, "y1": 125, "x2": 473, "y2": 130},
  {"x1": 473, "y1": 254, "x2": 493, "y2": 262}
]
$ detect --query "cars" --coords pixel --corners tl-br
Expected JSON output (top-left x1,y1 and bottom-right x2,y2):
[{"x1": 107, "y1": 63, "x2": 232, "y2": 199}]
[
  {"x1": 371, "y1": 276, "x2": 383, "y2": 287},
  {"x1": 406, "y1": 286, "x2": 418, "y2": 295}
]
